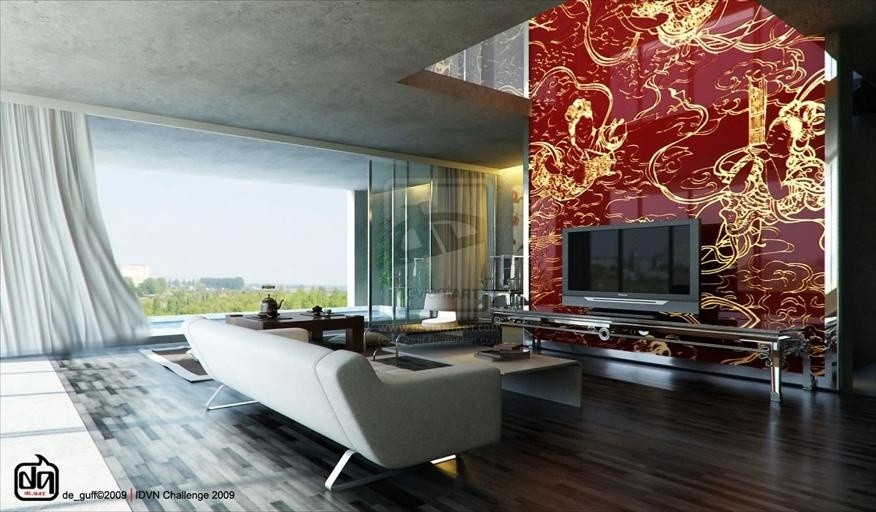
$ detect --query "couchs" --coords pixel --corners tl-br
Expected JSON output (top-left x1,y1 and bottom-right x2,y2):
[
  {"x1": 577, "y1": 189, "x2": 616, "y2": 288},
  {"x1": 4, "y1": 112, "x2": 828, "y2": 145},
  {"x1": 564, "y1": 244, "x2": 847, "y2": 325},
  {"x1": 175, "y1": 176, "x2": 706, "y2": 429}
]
[{"x1": 182, "y1": 315, "x2": 502, "y2": 492}]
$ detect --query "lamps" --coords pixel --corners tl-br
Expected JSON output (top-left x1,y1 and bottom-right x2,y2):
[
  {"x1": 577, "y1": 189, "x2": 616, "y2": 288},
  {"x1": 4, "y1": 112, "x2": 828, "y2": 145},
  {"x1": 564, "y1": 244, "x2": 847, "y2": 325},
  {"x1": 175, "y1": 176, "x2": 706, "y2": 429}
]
[{"x1": 421, "y1": 292, "x2": 459, "y2": 326}]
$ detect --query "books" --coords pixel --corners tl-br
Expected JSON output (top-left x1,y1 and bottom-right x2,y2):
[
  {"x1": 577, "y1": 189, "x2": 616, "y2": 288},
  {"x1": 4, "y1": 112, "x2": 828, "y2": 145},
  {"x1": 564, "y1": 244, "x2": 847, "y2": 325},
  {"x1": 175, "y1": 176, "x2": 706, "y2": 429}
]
[{"x1": 474, "y1": 342, "x2": 530, "y2": 363}]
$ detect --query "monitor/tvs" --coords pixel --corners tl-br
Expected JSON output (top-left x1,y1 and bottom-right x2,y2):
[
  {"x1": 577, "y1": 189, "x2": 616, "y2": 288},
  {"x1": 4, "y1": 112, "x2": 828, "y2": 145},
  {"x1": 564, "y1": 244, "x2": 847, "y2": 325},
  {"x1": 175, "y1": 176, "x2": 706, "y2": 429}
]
[{"x1": 561, "y1": 218, "x2": 702, "y2": 319}]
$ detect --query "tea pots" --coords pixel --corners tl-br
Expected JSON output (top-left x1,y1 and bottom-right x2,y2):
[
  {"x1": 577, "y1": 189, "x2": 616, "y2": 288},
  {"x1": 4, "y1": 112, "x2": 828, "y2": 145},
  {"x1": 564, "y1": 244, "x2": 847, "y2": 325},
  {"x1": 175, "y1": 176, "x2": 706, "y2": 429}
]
[{"x1": 259, "y1": 293, "x2": 285, "y2": 315}]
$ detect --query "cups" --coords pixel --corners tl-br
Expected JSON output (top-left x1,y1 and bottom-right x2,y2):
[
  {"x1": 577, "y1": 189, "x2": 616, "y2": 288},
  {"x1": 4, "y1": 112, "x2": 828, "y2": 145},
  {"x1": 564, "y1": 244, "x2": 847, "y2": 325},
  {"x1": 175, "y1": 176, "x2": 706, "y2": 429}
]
[
  {"x1": 323, "y1": 309, "x2": 331, "y2": 314},
  {"x1": 311, "y1": 305, "x2": 323, "y2": 312}
]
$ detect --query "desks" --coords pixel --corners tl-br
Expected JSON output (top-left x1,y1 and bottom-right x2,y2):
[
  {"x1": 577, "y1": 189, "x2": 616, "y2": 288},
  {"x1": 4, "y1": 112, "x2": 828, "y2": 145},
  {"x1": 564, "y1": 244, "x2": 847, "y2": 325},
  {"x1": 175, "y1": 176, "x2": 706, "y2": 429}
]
[
  {"x1": 491, "y1": 310, "x2": 816, "y2": 402},
  {"x1": 224, "y1": 314, "x2": 364, "y2": 356}
]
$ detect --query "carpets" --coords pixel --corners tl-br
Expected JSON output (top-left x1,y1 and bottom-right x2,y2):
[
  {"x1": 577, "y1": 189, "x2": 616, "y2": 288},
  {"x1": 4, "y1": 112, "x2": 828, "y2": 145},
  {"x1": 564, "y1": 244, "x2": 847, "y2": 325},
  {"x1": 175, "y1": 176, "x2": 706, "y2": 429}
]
[{"x1": 136, "y1": 347, "x2": 214, "y2": 382}]
